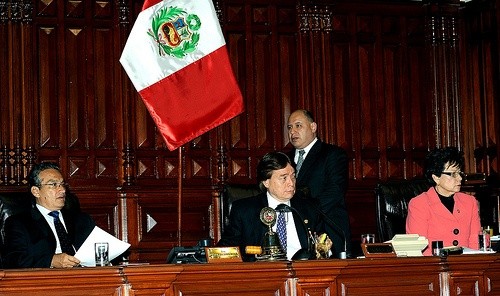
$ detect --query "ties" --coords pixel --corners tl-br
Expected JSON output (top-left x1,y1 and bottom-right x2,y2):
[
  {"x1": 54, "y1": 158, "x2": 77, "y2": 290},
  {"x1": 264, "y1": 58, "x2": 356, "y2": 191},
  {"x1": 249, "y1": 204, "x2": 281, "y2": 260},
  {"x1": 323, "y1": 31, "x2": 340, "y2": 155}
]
[
  {"x1": 47, "y1": 211, "x2": 76, "y2": 257},
  {"x1": 275, "y1": 212, "x2": 288, "y2": 254},
  {"x1": 296, "y1": 149, "x2": 306, "y2": 179}
]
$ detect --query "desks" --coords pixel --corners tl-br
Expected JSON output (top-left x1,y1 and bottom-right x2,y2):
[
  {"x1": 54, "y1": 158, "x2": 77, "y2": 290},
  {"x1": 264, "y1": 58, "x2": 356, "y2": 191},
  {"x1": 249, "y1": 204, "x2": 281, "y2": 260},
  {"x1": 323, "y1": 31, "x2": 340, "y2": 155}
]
[{"x1": 0, "y1": 251, "x2": 500, "y2": 296}]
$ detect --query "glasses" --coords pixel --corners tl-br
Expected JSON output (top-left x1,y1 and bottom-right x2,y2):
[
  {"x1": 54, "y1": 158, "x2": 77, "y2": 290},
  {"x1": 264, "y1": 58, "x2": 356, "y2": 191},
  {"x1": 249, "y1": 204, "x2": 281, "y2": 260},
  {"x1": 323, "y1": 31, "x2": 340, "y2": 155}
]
[
  {"x1": 40, "y1": 182, "x2": 69, "y2": 189},
  {"x1": 440, "y1": 171, "x2": 467, "y2": 179}
]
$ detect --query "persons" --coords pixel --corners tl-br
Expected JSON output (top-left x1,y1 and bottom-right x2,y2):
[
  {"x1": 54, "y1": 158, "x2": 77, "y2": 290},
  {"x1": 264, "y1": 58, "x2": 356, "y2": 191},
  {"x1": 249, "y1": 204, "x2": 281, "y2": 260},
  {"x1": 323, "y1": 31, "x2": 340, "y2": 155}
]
[
  {"x1": 4, "y1": 163, "x2": 94, "y2": 269},
  {"x1": 287, "y1": 110, "x2": 349, "y2": 259},
  {"x1": 216, "y1": 152, "x2": 325, "y2": 262},
  {"x1": 406, "y1": 154, "x2": 483, "y2": 256}
]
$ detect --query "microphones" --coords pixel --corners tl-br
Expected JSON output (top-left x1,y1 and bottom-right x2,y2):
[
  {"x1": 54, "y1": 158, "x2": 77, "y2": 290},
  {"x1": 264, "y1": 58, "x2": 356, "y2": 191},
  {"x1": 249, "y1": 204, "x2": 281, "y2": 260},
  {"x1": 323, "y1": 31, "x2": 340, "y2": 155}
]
[{"x1": 299, "y1": 191, "x2": 347, "y2": 253}]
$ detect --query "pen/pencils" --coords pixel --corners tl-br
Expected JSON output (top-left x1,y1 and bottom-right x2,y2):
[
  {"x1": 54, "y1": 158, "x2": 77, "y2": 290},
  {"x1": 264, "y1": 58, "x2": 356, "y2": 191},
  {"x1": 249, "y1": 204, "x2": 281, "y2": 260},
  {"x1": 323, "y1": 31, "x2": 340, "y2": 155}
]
[{"x1": 72, "y1": 244, "x2": 82, "y2": 266}]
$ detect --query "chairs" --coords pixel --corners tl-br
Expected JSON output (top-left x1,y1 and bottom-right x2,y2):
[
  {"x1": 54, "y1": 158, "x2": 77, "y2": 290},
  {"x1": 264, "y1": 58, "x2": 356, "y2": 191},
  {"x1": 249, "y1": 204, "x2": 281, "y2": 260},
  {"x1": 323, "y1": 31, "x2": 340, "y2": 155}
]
[
  {"x1": 0, "y1": 187, "x2": 81, "y2": 249},
  {"x1": 219, "y1": 182, "x2": 263, "y2": 234},
  {"x1": 374, "y1": 180, "x2": 421, "y2": 243}
]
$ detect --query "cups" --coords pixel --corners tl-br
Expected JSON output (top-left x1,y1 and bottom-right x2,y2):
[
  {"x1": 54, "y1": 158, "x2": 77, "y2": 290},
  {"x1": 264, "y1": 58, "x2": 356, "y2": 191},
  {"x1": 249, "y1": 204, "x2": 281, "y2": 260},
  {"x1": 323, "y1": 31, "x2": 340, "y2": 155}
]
[
  {"x1": 361, "y1": 234, "x2": 375, "y2": 243},
  {"x1": 198, "y1": 239, "x2": 213, "y2": 247},
  {"x1": 478, "y1": 230, "x2": 491, "y2": 252},
  {"x1": 432, "y1": 241, "x2": 443, "y2": 255},
  {"x1": 94, "y1": 242, "x2": 110, "y2": 266}
]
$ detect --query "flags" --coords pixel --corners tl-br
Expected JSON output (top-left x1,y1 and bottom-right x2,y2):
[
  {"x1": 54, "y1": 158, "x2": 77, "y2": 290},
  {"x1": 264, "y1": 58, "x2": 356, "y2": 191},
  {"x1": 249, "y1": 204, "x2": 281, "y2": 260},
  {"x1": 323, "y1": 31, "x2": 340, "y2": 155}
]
[{"x1": 119, "y1": 0, "x2": 246, "y2": 151}]
